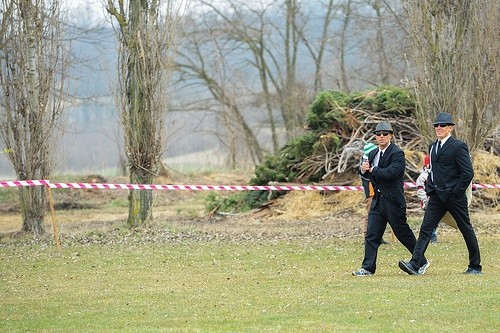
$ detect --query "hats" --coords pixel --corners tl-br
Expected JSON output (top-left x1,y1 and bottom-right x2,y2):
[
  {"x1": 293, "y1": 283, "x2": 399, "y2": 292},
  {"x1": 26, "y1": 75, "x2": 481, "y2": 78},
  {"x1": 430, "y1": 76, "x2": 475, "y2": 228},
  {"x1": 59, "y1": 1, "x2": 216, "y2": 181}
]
[
  {"x1": 363, "y1": 143, "x2": 377, "y2": 156},
  {"x1": 425, "y1": 156, "x2": 430, "y2": 164},
  {"x1": 372, "y1": 122, "x2": 393, "y2": 134},
  {"x1": 432, "y1": 113, "x2": 456, "y2": 125}
]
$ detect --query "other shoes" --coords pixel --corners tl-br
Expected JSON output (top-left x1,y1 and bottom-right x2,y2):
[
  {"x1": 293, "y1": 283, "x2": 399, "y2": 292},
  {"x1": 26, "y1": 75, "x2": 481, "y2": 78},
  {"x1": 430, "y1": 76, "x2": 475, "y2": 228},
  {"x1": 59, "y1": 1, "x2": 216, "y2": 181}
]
[
  {"x1": 381, "y1": 240, "x2": 388, "y2": 244},
  {"x1": 399, "y1": 261, "x2": 418, "y2": 276},
  {"x1": 464, "y1": 269, "x2": 479, "y2": 274}
]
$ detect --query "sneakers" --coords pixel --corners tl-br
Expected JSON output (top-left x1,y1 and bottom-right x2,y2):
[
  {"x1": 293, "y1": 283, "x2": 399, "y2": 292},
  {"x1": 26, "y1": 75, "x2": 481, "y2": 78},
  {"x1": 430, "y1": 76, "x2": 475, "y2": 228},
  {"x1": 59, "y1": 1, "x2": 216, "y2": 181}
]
[
  {"x1": 418, "y1": 261, "x2": 429, "y2": 274},
  {"x1": 352, "y1": 268, "x2": 372, "y2": 276}
]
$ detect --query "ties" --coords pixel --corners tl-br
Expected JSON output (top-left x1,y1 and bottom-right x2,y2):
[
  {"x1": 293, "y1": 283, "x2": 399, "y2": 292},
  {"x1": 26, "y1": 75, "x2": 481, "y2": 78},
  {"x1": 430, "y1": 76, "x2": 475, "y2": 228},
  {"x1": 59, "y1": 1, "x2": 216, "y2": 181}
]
[
  {"x1": 378, "y1": 152, "x2": 383, "y2": 165},
  {"x1": 437, "y1": 140, "x2": 442, "y2": 154}
]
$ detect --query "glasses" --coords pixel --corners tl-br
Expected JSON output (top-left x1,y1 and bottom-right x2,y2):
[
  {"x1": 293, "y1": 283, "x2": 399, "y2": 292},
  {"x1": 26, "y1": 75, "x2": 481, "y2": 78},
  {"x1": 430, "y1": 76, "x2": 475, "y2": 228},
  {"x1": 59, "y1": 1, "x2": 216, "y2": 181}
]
[
  {"x1": 375, "y1": 132, "x2": 389, "y2": 136},
  {"x1": 434, "y1": 124, "x2": 451, "y2": 127}
]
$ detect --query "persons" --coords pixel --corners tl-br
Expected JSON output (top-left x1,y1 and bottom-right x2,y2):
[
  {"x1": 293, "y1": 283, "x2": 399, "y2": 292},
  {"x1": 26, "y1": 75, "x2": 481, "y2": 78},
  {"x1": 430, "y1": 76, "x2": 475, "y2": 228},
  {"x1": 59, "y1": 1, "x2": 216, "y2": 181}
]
[
  {"x1": 359, "y1": 143, "x2": 439, "y2": 243},
  {"x1": 398, "y1": 113, "x2": 483, "y2": 275},
  {"x1": 352, "y1": 121, "x2": 431, "y2": 276}
]
